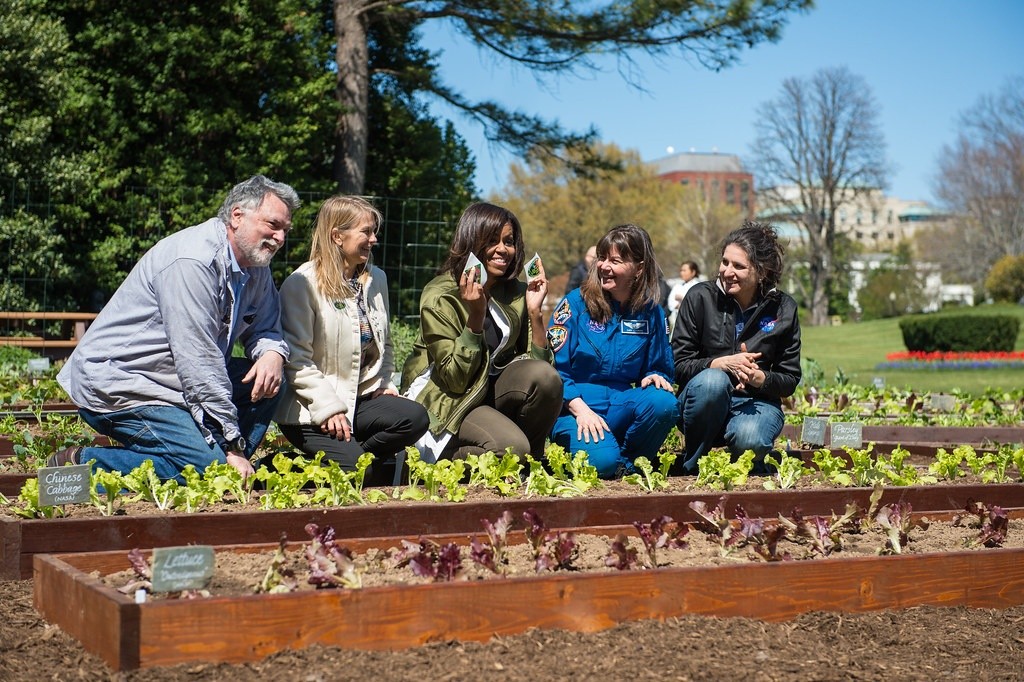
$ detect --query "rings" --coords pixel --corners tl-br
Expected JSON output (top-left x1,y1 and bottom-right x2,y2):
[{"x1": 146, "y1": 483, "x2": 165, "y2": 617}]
[{"x1": 736, "y1": 371, "x2": 742, "y2": 375}]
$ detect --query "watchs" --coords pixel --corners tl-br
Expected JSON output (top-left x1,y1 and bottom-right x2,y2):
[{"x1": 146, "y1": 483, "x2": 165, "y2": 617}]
[{"x1": 223, "y1": 435, "x2": 247, "y2": 453}]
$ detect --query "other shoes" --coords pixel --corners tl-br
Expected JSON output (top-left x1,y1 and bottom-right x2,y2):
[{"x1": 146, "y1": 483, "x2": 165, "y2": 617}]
[{"x1": 48, "y1": 445, "x2": 83, "y2": 467}]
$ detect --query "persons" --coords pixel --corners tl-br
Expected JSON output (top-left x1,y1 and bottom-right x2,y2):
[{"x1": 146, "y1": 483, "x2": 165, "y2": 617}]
[
  {"x1": 668, "y1": 262, "x2": 700, "y2": 335},
  {"x1": 258, "y1": 195, "x2": 430, "y2": 487},
  {"x1": 669, "y1": 224, "x2": 802, "y2": 473},
  {"x1": 48, "y1": 172, "x2": 300, "y2": 498},
  {"x1": 402, "y1": 202, "x2": 564, "y2": 483},
  {"x1": 565, "y1": 246, "x2": 598, "y2": 296},
  {"x1": 552, "y1": 226, "x2": 680, "y2": 480}
]
